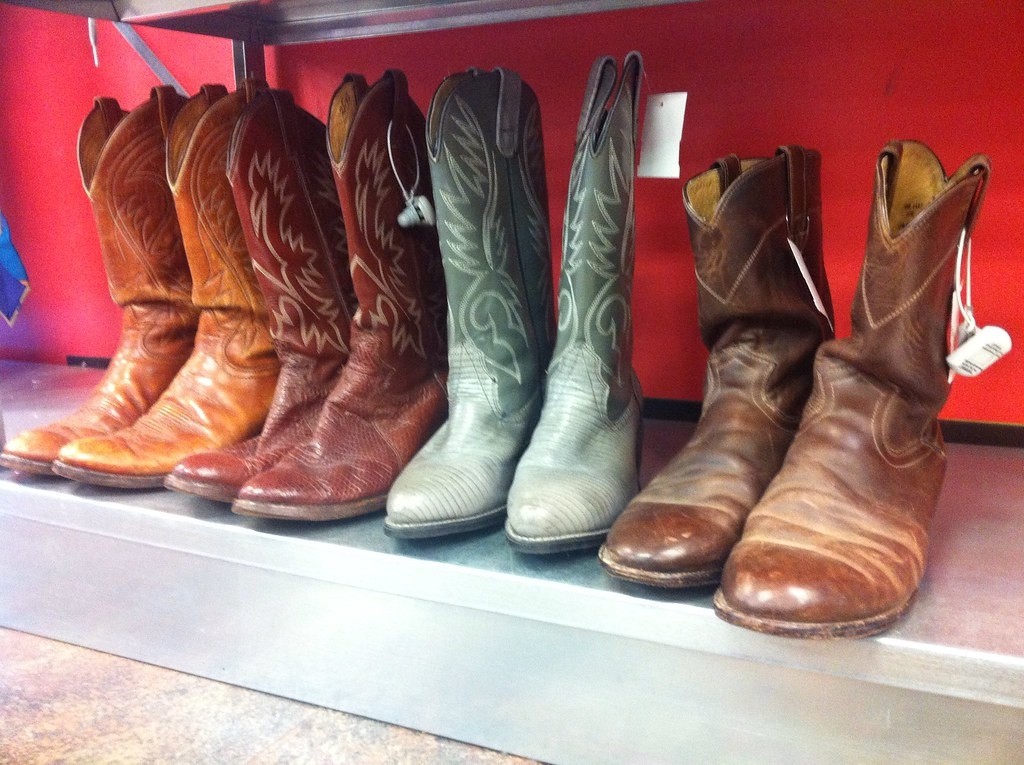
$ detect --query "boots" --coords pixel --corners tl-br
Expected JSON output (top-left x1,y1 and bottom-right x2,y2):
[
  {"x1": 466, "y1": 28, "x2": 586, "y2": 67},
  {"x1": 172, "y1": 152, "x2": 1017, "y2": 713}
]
[
  {"x1": 230, "y1": 67, "x2": 450, "y2": 519},
  {"x1": 382, "y1": 65, "x2": 554, "y2": 540},
  {"x1": 504, "y1": 49, "x2": 643, "y2": 553},
  {"x1": 161, "y1": 74, "x2": 356, "y2": 505},
  {"x1": 712, "y1": 134, "x2": 991, "y2": 639},
  {"x1": 0, "y1": 84, "x2": 201, "y2": 476},
  {"x1": 50, "y1": 82, "x2": 281, "y2": 489},
  {"x1": 597, "y1": 143, "x2": 836, "y2": 589}
]
